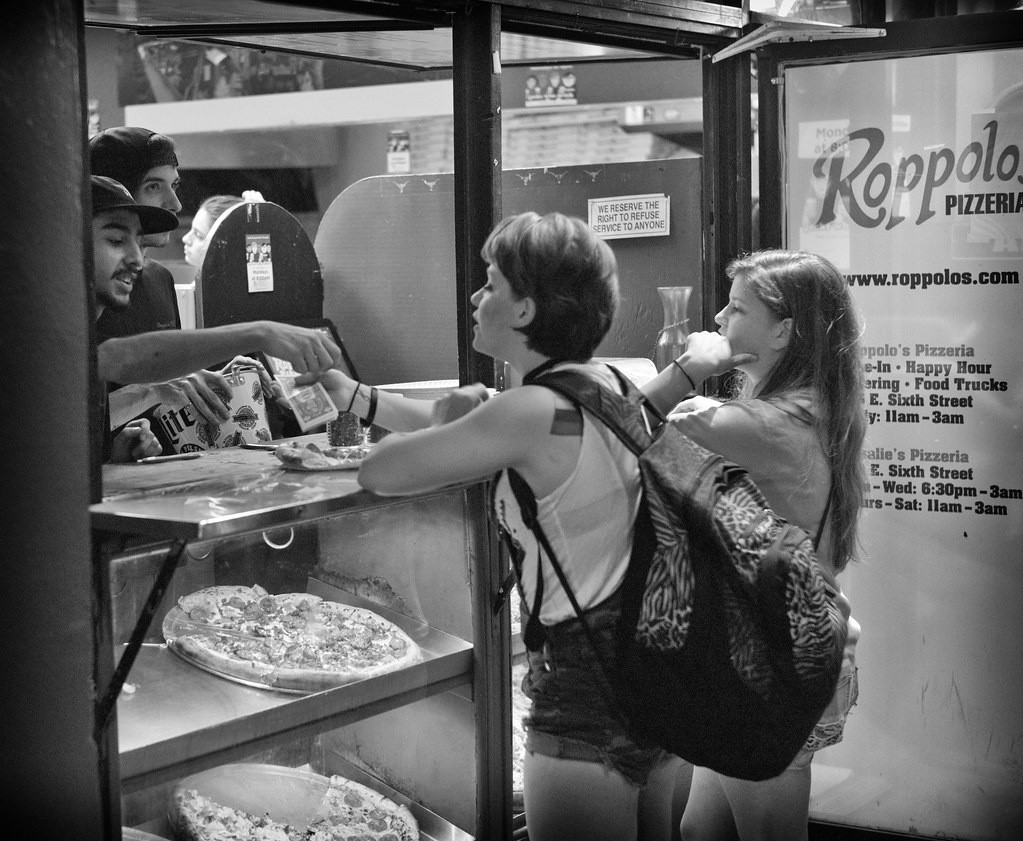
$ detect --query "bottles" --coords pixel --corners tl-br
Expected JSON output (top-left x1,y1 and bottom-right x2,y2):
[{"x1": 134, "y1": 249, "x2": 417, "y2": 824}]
[{"x1": 658, "y1": 286, "x2": 693, "y2": 371}]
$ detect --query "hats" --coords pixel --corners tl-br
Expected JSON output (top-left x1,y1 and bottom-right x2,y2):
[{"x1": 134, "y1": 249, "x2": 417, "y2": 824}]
[
  {"x1": 88, "y1": 126, "x2": 178, "y2": 170},
  {"x1": 91, "y1": 174, "x2": 179, "y2": 235}
]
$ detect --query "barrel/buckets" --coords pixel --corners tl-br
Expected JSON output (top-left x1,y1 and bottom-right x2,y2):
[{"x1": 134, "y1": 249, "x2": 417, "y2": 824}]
[{"x1": 152, "y1": 361, "x2": 272, "y2": 453}]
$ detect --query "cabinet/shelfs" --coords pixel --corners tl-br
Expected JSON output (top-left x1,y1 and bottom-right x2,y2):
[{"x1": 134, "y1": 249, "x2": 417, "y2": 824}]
[{"x1": 91, "y1": 432, "x2": 526, "y2": 841}]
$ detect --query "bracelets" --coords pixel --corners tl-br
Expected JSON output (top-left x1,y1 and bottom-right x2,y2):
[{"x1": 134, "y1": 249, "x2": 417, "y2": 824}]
[
  {"x1": 673, "y1": 360, "x2": 696, "y2": 393},
  {"x1": 360, "y1": 387, "x2": 378, "y2": 429},
  {"x1": 348, "y1": 381, "x2": 361, "y2": 412}
]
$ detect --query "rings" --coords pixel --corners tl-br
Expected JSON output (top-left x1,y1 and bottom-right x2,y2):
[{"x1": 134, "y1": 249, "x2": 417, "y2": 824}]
[{"x1": 185, "y1": 402, "x2": 192, "y2": 409}]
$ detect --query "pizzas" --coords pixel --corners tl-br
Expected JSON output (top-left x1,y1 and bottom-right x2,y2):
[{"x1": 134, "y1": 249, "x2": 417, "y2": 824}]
[
  {"x1": 173, "y1": 773, "x2": 421, "y2": 841},
  {"x1": 275, "y1": 441, "x2": 376, "y2": 470},
  {"x1": 171, "y1": 583, "x2": 421, "y2": 690}
]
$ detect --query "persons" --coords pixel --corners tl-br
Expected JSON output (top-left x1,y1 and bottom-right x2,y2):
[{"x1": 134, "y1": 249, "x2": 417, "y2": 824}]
[
  {"x1": 637, "y1": 249, "x2": 869, "y2": 841},
  {"x1": 318, "y1": 210, "x2": 681, "y2": 840},
  {"x1": 87, "y1": 127, "x2": 342, "y2": 463}
]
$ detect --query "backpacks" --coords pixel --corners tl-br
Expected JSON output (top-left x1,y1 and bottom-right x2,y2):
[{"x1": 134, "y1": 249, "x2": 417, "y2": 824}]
[{"x1": 506, "y1": 364, "x2": 852, "y2": 782}]
